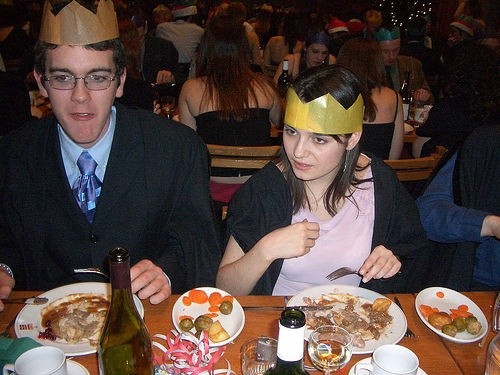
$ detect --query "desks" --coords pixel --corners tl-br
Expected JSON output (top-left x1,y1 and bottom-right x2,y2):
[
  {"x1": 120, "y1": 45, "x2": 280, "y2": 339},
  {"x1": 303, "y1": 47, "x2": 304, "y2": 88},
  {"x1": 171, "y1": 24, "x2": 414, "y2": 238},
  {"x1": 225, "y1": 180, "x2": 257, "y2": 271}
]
[{"x1": 1, "y1": 291, "x2": 500, "y2": 375}]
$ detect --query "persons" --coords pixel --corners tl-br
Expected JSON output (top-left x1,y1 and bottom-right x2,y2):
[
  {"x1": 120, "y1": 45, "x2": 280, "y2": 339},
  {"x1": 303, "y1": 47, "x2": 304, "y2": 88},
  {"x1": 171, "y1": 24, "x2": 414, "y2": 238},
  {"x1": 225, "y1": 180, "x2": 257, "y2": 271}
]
[
  {"x1": 417, "y1": 117, "x2": 500, "y2": 292},
  {"x1": 0, "y1": 0, "x2": 223, "y2": 312},
  {"x1": 216, "y1": 61, "x2": 425, "y2": 298},
  {"x1": 0, "y1": 0, "x2": 500, "y2": 202}
]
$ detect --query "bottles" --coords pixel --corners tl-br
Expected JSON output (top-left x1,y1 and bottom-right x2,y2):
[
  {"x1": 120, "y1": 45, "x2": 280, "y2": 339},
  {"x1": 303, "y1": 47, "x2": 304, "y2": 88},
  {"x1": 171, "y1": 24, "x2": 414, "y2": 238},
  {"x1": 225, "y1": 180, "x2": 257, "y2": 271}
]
[
  {"x1": 264, "y1": 307, "x2": 310, "y2": 375},
  {"x1": 277, "y1": 60, "x2": 291, "y2": 96},
  {"x1": 399, "y1": 71, "x2": 411, "y2": 122},
  {"x1": 96, "y1": 247, "x2": 155, "y2": 375}
]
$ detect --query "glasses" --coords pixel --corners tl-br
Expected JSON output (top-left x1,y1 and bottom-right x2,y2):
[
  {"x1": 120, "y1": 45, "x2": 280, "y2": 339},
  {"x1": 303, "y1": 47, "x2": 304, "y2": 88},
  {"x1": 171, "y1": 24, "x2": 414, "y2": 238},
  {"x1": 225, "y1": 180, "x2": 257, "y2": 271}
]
[{"x1": 42, "y1": 73, "x2": 116, "y2": 91}]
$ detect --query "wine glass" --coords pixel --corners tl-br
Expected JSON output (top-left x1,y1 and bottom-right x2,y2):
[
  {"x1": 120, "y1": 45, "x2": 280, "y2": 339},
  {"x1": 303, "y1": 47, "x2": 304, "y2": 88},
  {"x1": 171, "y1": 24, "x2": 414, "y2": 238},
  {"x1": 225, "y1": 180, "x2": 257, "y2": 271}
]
[
  {"x1": 307, "y1": 325, "x2": 353, "y2": 375},
  {"x1": 161, "y1": 96, "x2": 175, "y2": 120}
]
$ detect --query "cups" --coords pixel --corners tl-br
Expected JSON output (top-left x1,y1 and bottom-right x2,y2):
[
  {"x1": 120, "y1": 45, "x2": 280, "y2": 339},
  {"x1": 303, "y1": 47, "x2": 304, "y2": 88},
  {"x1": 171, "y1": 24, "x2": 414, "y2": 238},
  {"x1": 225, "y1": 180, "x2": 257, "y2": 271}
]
[
  {"x1": 3, "y1": 346, "x2": 67, "y2": 375},
  {"x1": 354, "y1": 344, "x2": 419, "y2": 375},
  {"x1": 492, "y1": 293, "x2": 500, "y2": 332},
  {"x1": 414, "y1": 105, "x2": 433, "y2": 123},
  {"x1": 241, "y1": 337, "x2": 278, "y2": 375},
  {"x1": 485, "y1": 333, "x2": 500, "y2": 375}
]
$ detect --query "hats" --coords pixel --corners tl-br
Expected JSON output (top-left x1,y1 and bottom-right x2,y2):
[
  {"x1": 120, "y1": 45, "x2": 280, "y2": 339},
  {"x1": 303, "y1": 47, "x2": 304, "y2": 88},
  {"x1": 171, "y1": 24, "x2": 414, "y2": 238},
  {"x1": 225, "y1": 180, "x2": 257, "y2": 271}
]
[
  {"x1": 171, "y1": 5, "x2": 198, "y2": 18},
  {"x1": 449, "y1": 19, "x2": 477, "y2": 38},
  {"x1": 328, "y1": 16, "x2": 349, "y2": 34}
]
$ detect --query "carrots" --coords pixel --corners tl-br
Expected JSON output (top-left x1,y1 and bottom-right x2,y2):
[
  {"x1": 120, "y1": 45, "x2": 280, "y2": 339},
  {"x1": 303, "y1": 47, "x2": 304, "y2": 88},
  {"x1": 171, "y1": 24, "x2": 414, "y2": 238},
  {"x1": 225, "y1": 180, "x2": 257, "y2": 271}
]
[
  {"x1": 420, "y1": 292, "x2": 473, "y2": 321},
  {"x1": 180, "y1": 290, "x2": 234, "y2": 320}
]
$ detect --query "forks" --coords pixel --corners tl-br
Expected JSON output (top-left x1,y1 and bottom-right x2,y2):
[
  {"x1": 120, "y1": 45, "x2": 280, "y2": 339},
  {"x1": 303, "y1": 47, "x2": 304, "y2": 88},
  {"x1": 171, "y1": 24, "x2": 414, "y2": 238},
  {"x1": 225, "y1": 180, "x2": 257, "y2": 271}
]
[
  {"x1": 74, "y1": 268, "x2": 109, "y2": 279},
  {"x1": 394, "y1": 296, "x2": 415, "y2": 338},
  {"x1": 326, "y1": 266, "x2": 363, "y2": 281}
]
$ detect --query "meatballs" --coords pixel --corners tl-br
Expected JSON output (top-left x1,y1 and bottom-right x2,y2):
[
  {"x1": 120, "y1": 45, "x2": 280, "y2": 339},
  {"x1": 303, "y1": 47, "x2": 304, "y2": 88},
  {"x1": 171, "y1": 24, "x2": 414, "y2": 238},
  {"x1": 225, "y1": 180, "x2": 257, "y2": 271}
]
[
  {"x1": 428, "y1": 312, "x2": 481, "y2": 337},
  {"x1": 220, "y1": 301, "x2": 233, "y2": 315},
  {"x1": 179, "y1": 316, "x2": 214, "y2": 332}
]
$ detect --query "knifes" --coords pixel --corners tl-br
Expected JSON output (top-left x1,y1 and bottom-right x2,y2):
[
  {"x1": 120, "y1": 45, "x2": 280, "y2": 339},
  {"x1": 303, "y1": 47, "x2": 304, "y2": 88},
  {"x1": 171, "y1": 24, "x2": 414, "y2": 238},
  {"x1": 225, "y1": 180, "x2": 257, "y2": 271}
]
[{"x1": 1, "y1": 298, "x2": 48, "y2": 305}]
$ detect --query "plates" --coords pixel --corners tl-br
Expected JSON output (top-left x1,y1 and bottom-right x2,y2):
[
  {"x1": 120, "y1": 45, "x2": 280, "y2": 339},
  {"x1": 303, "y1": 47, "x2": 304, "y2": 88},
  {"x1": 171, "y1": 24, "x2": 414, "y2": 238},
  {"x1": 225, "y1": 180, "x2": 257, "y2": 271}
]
[
  {"x1": 414, "y1": 286, "x2": 488, "y2": 344},
  {"x1": 14, "y1": 281, "x2": 144, "y2": 356},
  {"x1": 172, "y1": 286, "x2": 245, "y2": 346},
  {"x1": 10, "y1": 359, "x2": 90, "y2": 375},
  {"x1": 285, "y1": 285, "x2": 408, "y2": 354},
  {"x1": 348, "y1": 356, "x2": 427, "y2": 375}
]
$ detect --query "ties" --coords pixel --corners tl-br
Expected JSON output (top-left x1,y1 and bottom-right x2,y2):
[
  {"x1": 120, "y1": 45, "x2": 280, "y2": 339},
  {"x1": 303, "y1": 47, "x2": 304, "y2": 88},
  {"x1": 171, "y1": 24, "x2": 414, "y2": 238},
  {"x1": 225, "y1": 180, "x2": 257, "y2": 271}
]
[{"x1": 73, "y1": 152, "x2": 103, "y2": 225}]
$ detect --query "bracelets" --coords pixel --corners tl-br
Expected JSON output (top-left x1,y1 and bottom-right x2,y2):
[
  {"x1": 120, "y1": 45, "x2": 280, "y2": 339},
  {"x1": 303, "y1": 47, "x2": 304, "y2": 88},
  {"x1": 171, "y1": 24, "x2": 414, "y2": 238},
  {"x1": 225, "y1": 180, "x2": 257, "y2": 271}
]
[{"x1": 0, "y1": 263, "x2": 13, "y2": 277}]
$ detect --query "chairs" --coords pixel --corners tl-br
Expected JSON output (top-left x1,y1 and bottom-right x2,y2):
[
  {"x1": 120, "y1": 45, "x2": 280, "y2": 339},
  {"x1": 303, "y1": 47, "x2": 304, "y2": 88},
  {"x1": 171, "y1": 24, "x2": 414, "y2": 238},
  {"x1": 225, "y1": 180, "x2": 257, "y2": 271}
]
[{"x1": 206, "y1": 144, "x2": 282, "y2": 224}]
